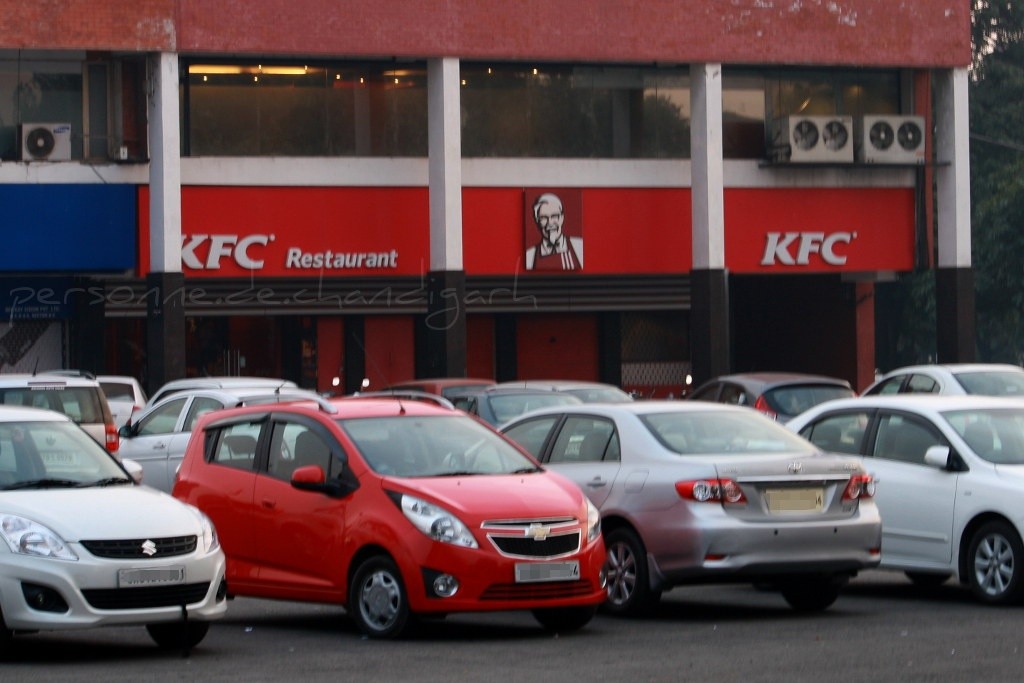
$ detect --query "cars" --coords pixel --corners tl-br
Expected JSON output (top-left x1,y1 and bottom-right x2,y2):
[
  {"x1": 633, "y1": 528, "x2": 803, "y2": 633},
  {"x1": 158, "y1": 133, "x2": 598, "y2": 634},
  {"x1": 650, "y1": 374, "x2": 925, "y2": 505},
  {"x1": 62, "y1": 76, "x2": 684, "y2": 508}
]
[
  {"x1": 61, "y1": 376, "x2": 148, "y2": 433},
  {"x1": 145, "y1": 377, "x2": 301, "y2": 434},
  {"x1": 167, "y1": 393, "x2": 613, "y2": 644},
  {"x1": 442, "y1": 403, "x2": 884, "y2": 615},
  {"x1": 857, "y1": 364, "x2": 1024, "y2": 447},
  {"x1": 659, "y1": 372, "x2": 871, "y2": 455},
  {"x1": 113, "y1": 387, "x2": 337, "y2": 497},
  {"x1": 746, "y1": 392, "x2": 1023, "y2": 608},
  {"x1": 0, "y1": 407, "x2": 230, "y2": 654},
  {"x1": 468, "y1": 377, "x2": 636, "y2": 458},
  {"x1": 373, "y1": 378, "x2": 498, "y2": 419}
]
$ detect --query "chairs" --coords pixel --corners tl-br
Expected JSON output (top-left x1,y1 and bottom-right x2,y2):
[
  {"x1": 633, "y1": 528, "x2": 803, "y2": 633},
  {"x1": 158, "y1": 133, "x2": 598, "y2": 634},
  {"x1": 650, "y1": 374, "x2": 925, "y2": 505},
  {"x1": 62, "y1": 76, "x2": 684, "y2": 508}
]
[
  {"x1": 295, "y1": 430, "x2": 342, "y2": 479},
  {"x1": 576, "y1": 431, "x2": 616, "y2": 461}
]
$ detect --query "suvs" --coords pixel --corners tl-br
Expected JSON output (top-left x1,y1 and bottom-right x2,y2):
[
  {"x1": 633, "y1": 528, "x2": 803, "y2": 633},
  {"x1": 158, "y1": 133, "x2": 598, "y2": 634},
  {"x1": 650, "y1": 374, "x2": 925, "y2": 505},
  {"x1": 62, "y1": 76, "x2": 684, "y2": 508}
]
[{"x1": 1, "y1": 371, "x2": 121, "y2": 483}]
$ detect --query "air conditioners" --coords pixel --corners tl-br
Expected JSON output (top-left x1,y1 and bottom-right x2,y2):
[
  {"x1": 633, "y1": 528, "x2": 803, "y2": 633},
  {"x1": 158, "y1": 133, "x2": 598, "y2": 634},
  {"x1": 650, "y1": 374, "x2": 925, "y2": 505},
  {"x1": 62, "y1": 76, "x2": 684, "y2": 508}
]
[
  {"x1": 16, "y1": 123, "x2": 70, "y2": 162},
  {"x1": 772, "y1": 115, "x2": 853, "y2": 164},
  {"x1": 854, "y1": 113, "x2": 925, "y2": 166}
]
